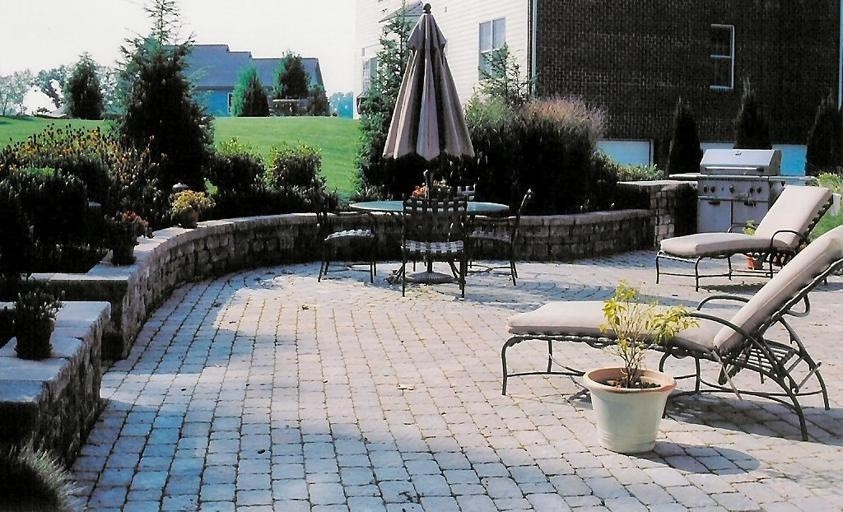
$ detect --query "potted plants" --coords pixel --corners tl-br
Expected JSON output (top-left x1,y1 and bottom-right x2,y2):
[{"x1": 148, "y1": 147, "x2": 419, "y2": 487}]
[
  {"x1": 736, "y1": 218, "x2": 767, "y2": 273},
  {"x1": 102, "y1": 207, "x2": 156, "y2": 266},
  {"x1": 162, "y1": 187, "x2": 216, "y2": 230},
  {"x1": 9, "y1": 283, "x2": 68, "y2": 359},
  {"x1": 576, "y1": 275, "x2": 704, "y2": 457}
]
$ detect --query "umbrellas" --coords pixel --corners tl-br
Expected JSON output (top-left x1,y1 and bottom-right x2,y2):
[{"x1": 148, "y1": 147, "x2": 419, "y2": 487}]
[{"x1": 382, "y1": 4, "x2": 476, "y2": 274}]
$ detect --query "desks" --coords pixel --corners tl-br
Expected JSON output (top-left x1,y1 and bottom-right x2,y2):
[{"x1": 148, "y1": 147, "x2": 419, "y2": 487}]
[{"x1": 348, "y1": 200, "x2": 511, "y2": 286}]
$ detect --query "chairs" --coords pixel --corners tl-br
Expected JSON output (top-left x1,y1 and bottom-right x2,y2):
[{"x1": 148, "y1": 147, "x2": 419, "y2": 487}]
[
  {"x1": 464, "y1": 187, "x2": 539, "y2": 288},
  {"x1": 410, "y1": 179, "x2": 479, "y2": 272},
  {"x1": 302, "y1": 186, "x2": 380, "y2": 284},
  {"x1": 398, "y1": 190, "x2": 469, "y2": 299},
  {"x1": 498, "y1": 218, "x2": 843, "y2": 447},
  {"x1": 650, "y1": 184, "x2": 837, "y2": 298}
]
